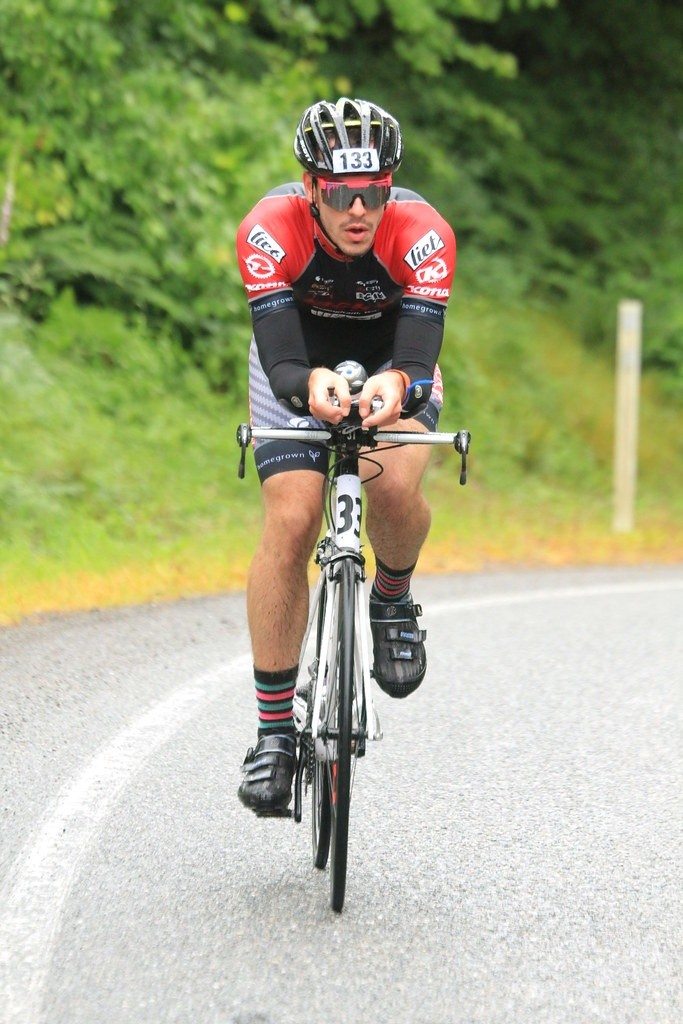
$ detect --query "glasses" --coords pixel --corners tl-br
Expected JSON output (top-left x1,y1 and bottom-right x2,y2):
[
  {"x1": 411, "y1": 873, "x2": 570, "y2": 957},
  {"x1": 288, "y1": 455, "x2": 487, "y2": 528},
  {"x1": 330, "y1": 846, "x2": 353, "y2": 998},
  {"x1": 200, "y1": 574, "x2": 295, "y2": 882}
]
[{"x1": 314, "y1": 172, "x2": 393, "y2": 212}]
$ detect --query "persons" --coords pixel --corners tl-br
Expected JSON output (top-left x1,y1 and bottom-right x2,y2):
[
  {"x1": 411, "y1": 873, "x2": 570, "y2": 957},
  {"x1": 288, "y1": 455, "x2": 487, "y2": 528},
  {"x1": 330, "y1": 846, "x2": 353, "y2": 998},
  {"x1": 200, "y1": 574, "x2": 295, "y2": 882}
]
[{"x1": 237, "y1": 96, "x2": 456, "y2": 811}]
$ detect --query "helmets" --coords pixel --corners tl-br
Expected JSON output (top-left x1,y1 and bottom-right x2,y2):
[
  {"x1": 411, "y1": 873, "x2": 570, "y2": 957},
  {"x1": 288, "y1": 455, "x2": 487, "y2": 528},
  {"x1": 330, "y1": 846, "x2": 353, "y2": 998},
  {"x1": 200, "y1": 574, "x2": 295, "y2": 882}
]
[{"x1": 294, "y1": 97, "x2": 403, "y2": 173}]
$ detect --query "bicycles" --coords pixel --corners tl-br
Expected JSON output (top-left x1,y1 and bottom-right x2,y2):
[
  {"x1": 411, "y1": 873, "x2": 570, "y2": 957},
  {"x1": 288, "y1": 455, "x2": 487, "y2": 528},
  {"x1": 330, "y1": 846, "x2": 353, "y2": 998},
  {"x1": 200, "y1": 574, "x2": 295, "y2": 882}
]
[{"x1": 236, "y1": 361, "x2": 471, "y2": 913}]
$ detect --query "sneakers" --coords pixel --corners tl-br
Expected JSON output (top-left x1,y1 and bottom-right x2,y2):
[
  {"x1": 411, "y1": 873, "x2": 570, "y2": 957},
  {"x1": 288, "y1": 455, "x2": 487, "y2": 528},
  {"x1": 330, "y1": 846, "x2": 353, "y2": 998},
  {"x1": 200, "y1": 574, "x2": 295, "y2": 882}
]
[
  {"x1": 369, "y1": 592, "x2": 427, "y2": 698},
  {"x1": 237, "y1": 728, "x2": 297, "y2": 812}
]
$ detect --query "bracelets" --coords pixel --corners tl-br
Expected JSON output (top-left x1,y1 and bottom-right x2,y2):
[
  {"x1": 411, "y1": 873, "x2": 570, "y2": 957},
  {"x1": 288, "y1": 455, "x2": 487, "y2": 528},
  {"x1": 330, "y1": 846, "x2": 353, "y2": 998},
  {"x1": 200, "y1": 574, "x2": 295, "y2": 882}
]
[{"x1": 386, "y1": 368, "x2": 411, "y2": 393}]
